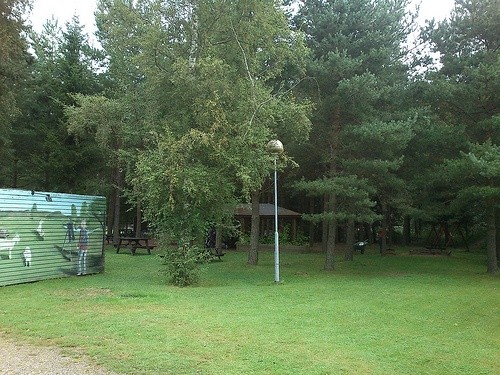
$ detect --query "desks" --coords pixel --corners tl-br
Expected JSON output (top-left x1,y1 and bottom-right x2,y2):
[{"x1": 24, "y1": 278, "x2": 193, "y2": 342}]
[
  {"x1": 202, "y1": 247, "x2": 222, "y2": 260},
  {"x1": 117, "y1": 237, "x2": 150, "y2": 256}
]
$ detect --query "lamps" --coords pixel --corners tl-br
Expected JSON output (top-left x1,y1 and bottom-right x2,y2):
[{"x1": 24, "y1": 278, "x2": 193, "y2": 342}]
[{"x1": 31, "y1": 190, "x2": 52, "y2": 202}]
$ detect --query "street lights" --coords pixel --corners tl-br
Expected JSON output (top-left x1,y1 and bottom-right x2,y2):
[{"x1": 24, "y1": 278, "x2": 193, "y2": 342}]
[{"x1": 266, "y1": 139, "x2": 284, "y2": 282}]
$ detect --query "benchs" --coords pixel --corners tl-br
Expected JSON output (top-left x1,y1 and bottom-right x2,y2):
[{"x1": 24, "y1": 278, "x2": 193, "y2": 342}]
[
  {"x1": 214, "y1": 254, "x2": 225, "y2": 256},
  {"x1": 114, "y1": 244, "x2": 137, "y2": 245},
  {"x1": 133, "y1": 246, "x2": 157, "y2": 247}
]
